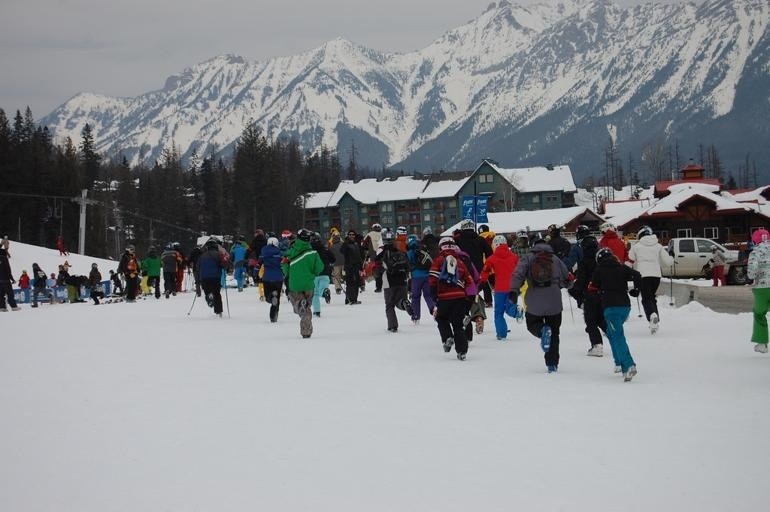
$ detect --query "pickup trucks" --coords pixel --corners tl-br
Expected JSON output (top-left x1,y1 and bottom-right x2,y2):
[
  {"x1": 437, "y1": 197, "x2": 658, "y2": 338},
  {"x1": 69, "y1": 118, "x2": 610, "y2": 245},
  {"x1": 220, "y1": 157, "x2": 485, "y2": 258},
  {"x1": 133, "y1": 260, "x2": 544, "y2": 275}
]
[{"x1": 660, "y1": 238, "x2": 751, "y2": 285}]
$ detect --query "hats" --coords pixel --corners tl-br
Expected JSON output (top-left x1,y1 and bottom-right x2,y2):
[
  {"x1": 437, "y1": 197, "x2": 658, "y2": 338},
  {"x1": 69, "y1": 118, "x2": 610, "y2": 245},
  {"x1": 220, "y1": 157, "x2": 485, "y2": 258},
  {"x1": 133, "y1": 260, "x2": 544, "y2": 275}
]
[
  {"x1": 92, "y1": 263, "x2": 97, "y2": 268},
  {"x1": 332, "y1": 236, "x2": 340, "y2": 244},
  {"x1": 438, "y1": 237, "x2": 455, "y2": 248},
  {"x1": 461, "y1": 219, "x2": 475, "y2": 230}
]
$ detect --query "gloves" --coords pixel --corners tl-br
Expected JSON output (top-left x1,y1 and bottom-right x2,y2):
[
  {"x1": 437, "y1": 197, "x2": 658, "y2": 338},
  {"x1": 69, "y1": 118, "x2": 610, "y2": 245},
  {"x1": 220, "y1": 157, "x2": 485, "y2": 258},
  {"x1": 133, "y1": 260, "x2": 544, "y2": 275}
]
[{"x1": 508, "y1": 291, "x2": 517, "y2": 304}]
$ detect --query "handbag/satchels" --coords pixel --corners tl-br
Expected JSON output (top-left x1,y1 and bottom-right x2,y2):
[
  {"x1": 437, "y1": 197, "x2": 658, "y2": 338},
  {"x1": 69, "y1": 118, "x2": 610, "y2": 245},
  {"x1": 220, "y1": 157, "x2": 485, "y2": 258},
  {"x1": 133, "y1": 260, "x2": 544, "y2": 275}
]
[
  {"x1": 439, "y1": 255, "x2": 460, "y2": 287},
  {"x1": 429, "y1": 285, "x2": 438, "y2": 304}
]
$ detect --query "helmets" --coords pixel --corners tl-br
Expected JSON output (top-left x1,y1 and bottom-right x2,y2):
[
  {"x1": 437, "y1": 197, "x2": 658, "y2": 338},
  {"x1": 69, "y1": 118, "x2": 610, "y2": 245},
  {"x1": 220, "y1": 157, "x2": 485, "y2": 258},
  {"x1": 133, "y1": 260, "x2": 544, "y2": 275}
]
[
  {"x1": 595, "y1": 247, "x2": 613, "y2": 264},
  {"x1": 491, "y1": 235, "x2": 507, "y2": 254},
  {"x1": 371, "y1": 224, "x2": 432, "y2": 246},
  {"x1": 254, "y1": 228, "x2": 320, "y2": 247},
  {"x1": 478, "y1": 225, "x2": 489, "y2": 234},
  {"x1": 636, "y1": 225, "x2": 653, "y2": 240},
  {"x1": 516, "y1": 224, "x2": 529, "y2": 237},
  {"x1": 548, "y1": 223, "x2": 615, "y2": 240},
  {"x1": 126, "y1": 244, "x2": 136, "y2": 251},
  {"x1": 751, "y1": 229, "x2": 770, "y2": 244},
  {"x1": 206, "y1": 237, "x2": 219, "y2": 246}
]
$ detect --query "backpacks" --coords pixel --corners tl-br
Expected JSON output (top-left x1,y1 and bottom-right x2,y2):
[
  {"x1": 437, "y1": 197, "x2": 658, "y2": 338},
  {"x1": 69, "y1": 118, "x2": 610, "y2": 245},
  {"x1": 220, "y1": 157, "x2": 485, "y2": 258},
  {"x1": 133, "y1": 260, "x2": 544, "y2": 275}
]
[
  {"x1": 379, "y1": 246, "x2": 409, "y2": 279},
  {"x1": 408, "y1": 246, "x2": 432, "y2": 270}
]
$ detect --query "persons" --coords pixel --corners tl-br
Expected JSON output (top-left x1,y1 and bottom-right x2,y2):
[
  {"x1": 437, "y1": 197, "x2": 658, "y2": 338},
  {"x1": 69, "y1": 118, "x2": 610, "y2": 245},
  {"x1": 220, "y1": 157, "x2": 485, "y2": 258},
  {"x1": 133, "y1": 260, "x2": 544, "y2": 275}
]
[
  {"x1": 230, "y1": 224, "x2": 384, "y2": 337},
  {"x1": 375, "y1": 221, "x2": 675, "y2": 382},
  {"x1": 711, "y1": 232, "x2": 770, "y2": 353},
  {"x1": 1, "y1": 235, "x2": 105, "y2": 311},
  {"x1": 109, "y1": 237, "x2": 230, "y2": 317}
]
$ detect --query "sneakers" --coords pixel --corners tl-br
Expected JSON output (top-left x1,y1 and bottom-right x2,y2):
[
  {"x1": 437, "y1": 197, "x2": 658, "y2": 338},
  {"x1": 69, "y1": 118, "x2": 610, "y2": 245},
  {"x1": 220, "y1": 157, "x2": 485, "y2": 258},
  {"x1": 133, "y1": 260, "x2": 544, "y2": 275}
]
[
  {"x1": 586, "y1": 344, "x2": 603, "y2": 357},
  {"x1": 614, "y1": 365, "x2": 637, "y2": 381},
  {"x1": 515, "y1": 305, "x2": 524, "y2": 324},
  {"x1": 388, "y1": 327, "x2": 397, "y2": 332},
  {"x1": 539, "y1": 325, "x2": 553, "y2": 353},
  {"x1": 1, "y1": 302, "x2": 38, "y2": 312},
  {"x1": 207, "y1": 293, "x2": 215, "y2": 307},
  {"x1": 443, "y1": 336, "x2": 467, "y2": 360},
  {"x1": 754, "y1": 343, "x2": 768, "y2": 353},
  {"x1": 476, "y1": 316, "x2": 484, "y2": 334},
  {"x1": 547, "y1": 364, "x2": 557, "y2": 373},
  {"x1": 401, "y1": 299, "x2": 420, "y2": 320},
  {"x1": 648, "y1": 312, "x2": 659, "y2": 334},
  {"x1": 166, "y1": 289, "x2": 177, "y2": 298},
  {"x1": 322, "y1": 284, "x2": 383, "y2": 304},
  {"x1": 272, "y1": 290, "x2": 321, "y2": 337}
]
